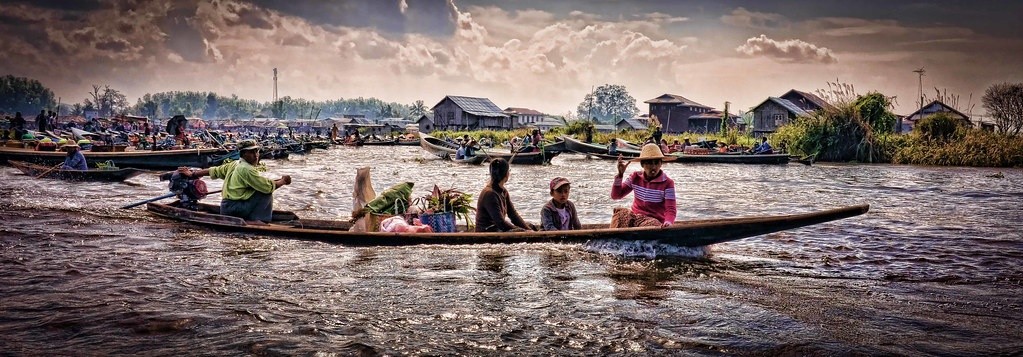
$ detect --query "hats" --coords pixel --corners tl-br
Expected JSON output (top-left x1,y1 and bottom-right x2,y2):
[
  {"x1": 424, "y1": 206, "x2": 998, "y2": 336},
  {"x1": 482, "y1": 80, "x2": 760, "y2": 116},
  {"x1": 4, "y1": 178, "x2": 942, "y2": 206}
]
[
  {"x1": 240, "y1": 140, "x2": 260, "y2": 152},
  {"x1": 61, "y1": 140, "x2": 80, "y2": 147},
  {"x1": 456, "y1": 136, "x2": 464, "y2": 139},
  {"x1": 628, "y1": 143, "x2": 677, "y2": 161},
  {"x1": 550, "y1": 177, "x2": 571, "y2": 189}
]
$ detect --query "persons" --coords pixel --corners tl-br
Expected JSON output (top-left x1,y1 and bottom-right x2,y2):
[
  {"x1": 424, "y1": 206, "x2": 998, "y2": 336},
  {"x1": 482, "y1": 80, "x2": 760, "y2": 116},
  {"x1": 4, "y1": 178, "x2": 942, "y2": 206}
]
[
  {"x1": 456, "y1": 134, "x2": 494, "y2": 160},
  {"x1": 541, "y1": 178, "x2": 582, "y2": 230},
  {"x1": 585, "y1": 124, "x2": 772, "y2": 160},
  {"x1": 0, "y1": 110, "x2": 270, "y2": 140},
  {"x1": 56, "y1": 139, "x2": 88, "y2": 170},
  {"x1": 510, "y1": 128, "x2": 542, "y2": 153},
  {"x1": 475, "y1": 159, "x2": 533, "y2": 233},
  {"x1": 278, "y1": 123, "x2": 414, "y2": 141},
  {"x1": 610, "y1": 143, "x2": 676, "y2": 232},
  {"x1": 178, "y1": 139, "x2": 292, "y2": 226}
]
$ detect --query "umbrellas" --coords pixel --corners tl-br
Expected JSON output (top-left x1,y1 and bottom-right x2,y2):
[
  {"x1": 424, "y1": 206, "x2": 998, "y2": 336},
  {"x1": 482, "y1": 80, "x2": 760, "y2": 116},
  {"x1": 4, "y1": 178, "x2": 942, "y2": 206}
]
[{"x1": 166, "y1": 114, "x2": 188, "y2": 140}]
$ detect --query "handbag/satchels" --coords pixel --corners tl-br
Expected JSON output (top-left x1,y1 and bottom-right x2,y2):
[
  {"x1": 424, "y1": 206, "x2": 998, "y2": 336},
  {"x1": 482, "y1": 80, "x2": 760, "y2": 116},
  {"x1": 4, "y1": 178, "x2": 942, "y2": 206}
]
[{"x1": 372, "y1": 197, "x2": 457, "y2": 233}]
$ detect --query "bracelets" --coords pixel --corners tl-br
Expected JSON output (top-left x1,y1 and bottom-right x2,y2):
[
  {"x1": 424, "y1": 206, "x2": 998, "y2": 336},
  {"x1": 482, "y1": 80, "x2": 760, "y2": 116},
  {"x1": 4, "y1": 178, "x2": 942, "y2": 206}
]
[{"x1": 191, "y1": 169, "x2": 199, "y2": 179}]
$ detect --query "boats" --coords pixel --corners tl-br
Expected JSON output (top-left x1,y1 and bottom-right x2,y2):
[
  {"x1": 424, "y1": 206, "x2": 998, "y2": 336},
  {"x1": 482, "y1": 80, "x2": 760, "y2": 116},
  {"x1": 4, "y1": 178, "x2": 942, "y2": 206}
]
[
  {"x1": 145, "y1": 168, "x2": 872, "y2": 245},
  {"x1": 0, "y1": 119, "x2": 419, "y2": 165},
  {"x1": 7, "y1": 159, "x2": 142, "y2": 181},
  {"x1": 554, "y1": 135, "x2": 789, "y2": 167},
  {"x1": 419, "y1": 131, "x2": 565, "y2": 165}
]
